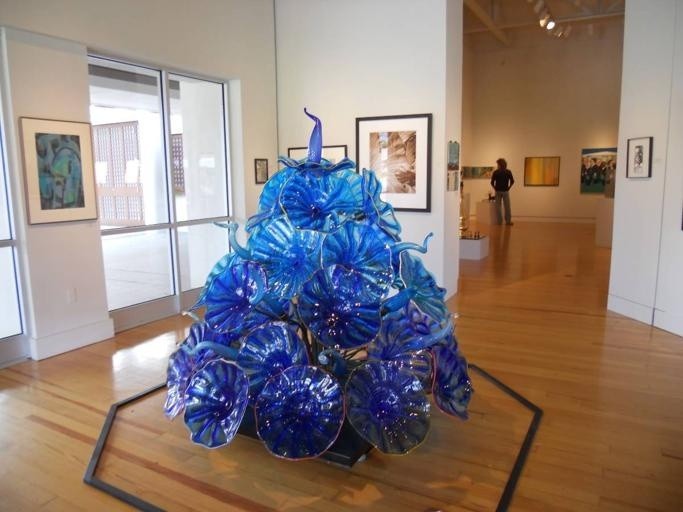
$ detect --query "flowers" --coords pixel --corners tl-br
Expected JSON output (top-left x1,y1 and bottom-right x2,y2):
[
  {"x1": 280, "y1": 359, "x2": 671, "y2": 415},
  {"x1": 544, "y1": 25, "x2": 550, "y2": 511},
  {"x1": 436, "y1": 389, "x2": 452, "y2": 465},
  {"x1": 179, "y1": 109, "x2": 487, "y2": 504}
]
[{"x1": 164, "y1": 106, "x2": 474, "y2": 463}]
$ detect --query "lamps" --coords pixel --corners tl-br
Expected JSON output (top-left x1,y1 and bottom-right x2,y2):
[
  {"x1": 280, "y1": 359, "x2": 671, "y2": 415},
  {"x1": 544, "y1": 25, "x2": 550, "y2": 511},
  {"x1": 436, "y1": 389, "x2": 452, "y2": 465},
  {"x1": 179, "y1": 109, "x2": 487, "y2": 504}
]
[{"x1": 532, "y1": 1, "x2": 573, "y2": 41}]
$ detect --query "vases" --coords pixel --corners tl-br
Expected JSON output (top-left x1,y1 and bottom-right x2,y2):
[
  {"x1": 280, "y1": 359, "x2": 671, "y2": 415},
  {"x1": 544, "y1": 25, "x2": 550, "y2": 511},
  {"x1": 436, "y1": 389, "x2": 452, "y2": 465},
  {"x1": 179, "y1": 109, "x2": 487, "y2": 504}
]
[{"x1": 237, "y1": 400, "x2": 373, "y2": 468}]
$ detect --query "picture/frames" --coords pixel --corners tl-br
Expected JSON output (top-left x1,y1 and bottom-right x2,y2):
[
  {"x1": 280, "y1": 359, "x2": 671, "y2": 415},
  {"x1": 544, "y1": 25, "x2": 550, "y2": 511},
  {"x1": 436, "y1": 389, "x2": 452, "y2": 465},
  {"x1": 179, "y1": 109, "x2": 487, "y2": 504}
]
[
  {"x1": 17, "y1": 115, "x2": 98, "y2": 227},
  {"x1": 625, "y1": 136, "x2": 653, "y2": 179},
  {"x1": 253, "y1": 144, "x2": 348, "y2": 185},
  {"x1": 353, "y1": 113, "x2": 431, "y2": 213}
]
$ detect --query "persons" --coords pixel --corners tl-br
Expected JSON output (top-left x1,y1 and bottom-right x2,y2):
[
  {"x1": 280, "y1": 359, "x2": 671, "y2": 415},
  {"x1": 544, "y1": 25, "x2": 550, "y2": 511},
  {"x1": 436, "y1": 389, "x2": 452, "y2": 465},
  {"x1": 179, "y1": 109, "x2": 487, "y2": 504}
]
[
  {"x1": 581, "y1": 157, "x2": 617, "y2": 186},
  {"x1": 36, "y1": 135, "x2": 84, "y2": 211},
  {"x1": 490, "y1": 158, "x2": 514, "y2": 226}
]
